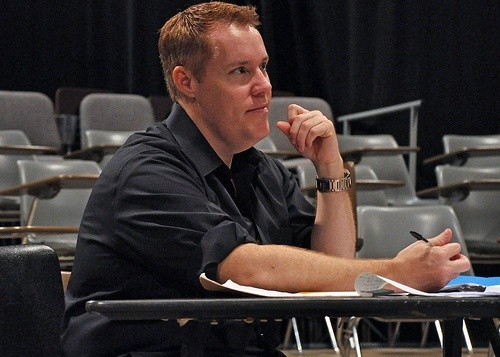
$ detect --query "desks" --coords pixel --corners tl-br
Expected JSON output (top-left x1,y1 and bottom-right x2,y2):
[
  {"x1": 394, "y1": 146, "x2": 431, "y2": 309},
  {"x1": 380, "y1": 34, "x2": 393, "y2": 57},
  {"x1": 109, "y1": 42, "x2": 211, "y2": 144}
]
[{"x1": 86, "y1": 292, "x2": 500, "y2": 357}]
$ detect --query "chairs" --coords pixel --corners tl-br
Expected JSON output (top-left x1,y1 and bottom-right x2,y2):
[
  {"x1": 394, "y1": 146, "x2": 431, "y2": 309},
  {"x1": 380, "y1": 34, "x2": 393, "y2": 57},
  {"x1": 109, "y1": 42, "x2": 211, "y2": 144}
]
[{"x1": 0, "y1": 88, "x2": 500, "y2": 357}]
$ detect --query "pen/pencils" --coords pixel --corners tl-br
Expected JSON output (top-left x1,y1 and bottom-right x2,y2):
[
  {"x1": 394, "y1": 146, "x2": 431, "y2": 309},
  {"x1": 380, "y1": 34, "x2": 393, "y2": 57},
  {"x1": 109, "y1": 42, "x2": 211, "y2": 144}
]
[{"x1": 409, "y1": 229, "x2": 430, "y2": 243}]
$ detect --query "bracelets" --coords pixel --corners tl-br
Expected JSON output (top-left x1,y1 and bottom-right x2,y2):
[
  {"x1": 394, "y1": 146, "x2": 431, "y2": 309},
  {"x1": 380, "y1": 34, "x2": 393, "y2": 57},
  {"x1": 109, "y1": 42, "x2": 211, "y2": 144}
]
[{"x1": 315, "y1": 169, "x2": 352, "y2": 192}]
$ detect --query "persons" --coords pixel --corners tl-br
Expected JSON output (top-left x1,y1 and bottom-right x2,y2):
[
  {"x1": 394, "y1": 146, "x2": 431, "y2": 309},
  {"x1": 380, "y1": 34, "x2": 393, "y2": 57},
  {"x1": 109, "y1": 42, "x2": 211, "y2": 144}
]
[{"x1": 61, "y1": 1, "x2": 471, "y2": 357}]
